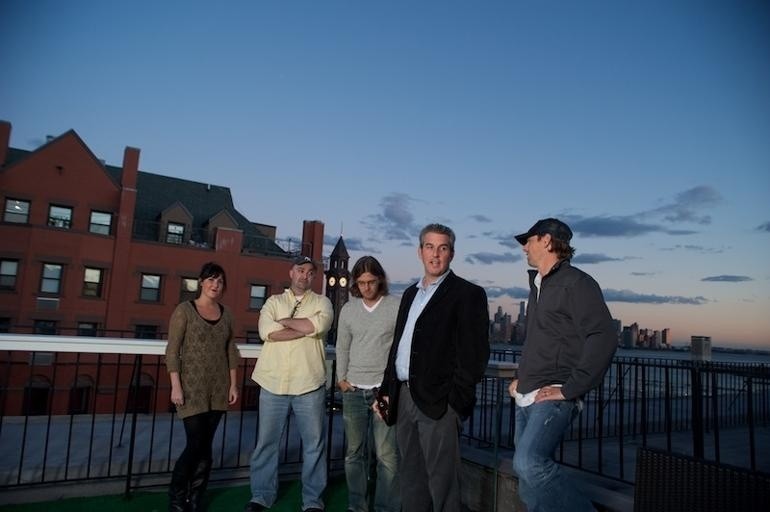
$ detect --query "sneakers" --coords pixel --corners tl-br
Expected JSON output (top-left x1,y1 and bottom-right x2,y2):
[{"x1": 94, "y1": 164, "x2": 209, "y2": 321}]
[{"x1": 244, "y1": 502, "x2": 268, "y2": 511}]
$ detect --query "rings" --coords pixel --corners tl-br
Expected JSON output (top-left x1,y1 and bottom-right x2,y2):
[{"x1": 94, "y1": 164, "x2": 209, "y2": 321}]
[{"x1": 544, "y1": 391, "x2": 548, "y2": 396}]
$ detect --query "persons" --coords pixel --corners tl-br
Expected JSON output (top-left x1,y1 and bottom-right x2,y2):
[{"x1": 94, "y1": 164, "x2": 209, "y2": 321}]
[
  {"x1": 165, "y1": 261, "x2": 242, "y2": 512},
  {"x1": 335, "y1": 255, "x2": 403, "y2": 511},
  {"x1": 242, "y1": 254, "x2": 335, "y2": 512},
  {"x1": 371, "y1": 222, "x2": 492, "y2": 511},
  {"x1": 507, "y1": 216, "x2": 619, "y2": 512}
]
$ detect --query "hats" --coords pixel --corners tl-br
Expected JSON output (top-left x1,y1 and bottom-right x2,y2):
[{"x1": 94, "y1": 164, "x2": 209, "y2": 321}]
[
  {"x1": 514, "y1": 218, "x2": 572, "y2": 245},
  {"x1": 290, "y1": 256, "x2": 317, "y2": 271}
]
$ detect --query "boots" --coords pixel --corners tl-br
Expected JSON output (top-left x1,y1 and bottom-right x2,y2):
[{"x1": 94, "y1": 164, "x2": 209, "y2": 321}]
[{"x1": 169, "y1": 459, "x2": 212, "y2": 512}]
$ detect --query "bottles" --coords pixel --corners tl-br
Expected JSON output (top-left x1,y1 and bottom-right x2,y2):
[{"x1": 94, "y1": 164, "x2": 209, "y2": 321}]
[{"x1": 372, "y1": 385, "x2": 395, "y2": 426}]
